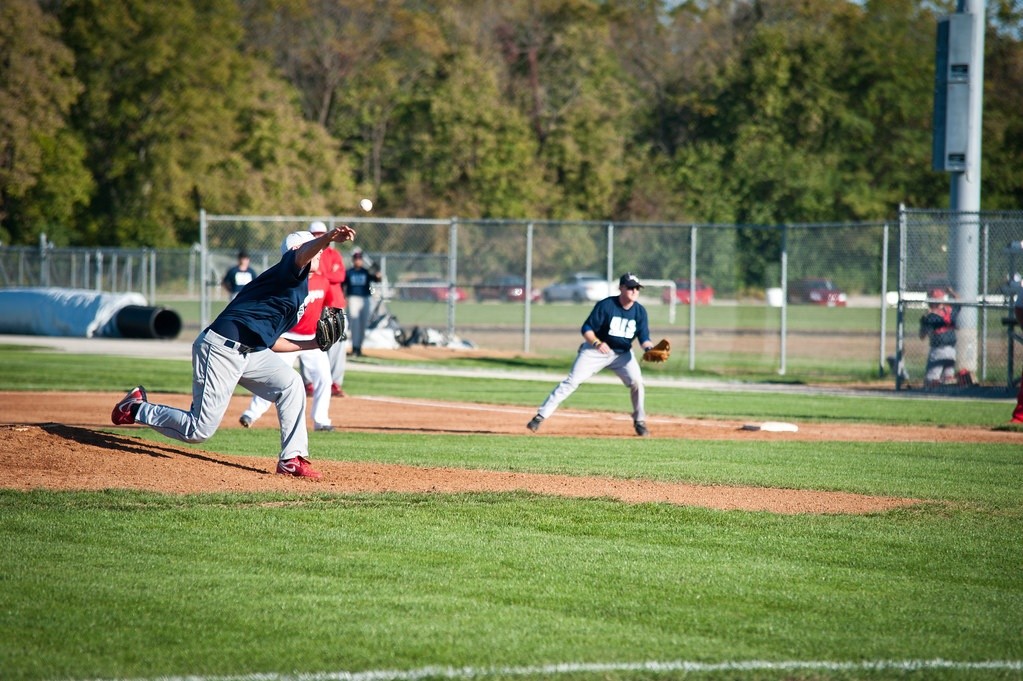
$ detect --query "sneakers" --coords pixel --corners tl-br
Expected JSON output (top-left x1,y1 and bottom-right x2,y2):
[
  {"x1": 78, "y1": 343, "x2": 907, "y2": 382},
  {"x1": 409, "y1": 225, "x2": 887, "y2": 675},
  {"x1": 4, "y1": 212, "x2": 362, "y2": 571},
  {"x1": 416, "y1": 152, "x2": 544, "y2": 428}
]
[
  {"x1": 111, "y1": 385, "x2": 147, "y2": 425},
  {"x1": 276, "y1": 456, "x2": 322, "y2": 480}
]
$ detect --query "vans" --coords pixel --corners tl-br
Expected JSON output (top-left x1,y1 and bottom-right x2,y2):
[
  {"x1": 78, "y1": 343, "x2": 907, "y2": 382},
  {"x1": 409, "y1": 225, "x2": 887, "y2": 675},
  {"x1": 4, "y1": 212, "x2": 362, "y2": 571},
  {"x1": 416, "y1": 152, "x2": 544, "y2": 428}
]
[{"x1": 791, "y1": 276, "x2": 847, "y2": 305}]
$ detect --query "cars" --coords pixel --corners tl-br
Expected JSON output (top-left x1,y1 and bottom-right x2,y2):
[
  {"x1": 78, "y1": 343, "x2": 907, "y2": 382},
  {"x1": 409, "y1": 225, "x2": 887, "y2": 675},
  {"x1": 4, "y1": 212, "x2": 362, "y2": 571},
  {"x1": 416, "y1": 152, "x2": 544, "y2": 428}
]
[
  {"x1": 395, "y1": 278, "x2": 464, "y2": 303},
  {"x1": 664, "y1": 278, "x2": 715, "y2": 304},
  {"x1": 908, "y1": 273, "x2": 950, "y2": 292},
  {"x1": 474, "y1": 271, "x2": 541, "y2": 304},
  {"x1": 543, "y1": 272, "x2": 608, "y2": 302}
]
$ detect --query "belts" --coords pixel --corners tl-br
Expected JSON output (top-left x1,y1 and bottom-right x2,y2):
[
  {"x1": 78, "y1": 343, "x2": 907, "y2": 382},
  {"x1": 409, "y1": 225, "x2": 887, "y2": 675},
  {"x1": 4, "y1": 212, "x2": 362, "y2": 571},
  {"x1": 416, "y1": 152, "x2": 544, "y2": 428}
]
[{"x1": 204, "y1": 327, "x2": 252, "y2": 357}]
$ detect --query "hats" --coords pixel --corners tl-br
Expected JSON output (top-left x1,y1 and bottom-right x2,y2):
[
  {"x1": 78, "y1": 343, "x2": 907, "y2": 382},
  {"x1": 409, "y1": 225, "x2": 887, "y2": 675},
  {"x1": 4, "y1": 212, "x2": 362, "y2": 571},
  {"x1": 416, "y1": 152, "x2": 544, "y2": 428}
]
[
  {"x1": 351, "y1": 245, "x2": 362, "y2": 256},
  {"x1": 309, "y1": 221, "x2": 327, "y2": 233},
  {"x1": 620, "y1": 272, "x2": 645, "y2": 289},
  {"x1": 280, "y1": 231, "x2": 316, "y2": 254}
]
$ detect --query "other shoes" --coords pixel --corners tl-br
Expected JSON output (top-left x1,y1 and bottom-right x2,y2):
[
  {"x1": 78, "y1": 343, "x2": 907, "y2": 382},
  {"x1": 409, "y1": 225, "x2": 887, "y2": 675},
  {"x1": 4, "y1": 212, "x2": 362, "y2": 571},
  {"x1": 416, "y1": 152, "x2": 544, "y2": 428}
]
[
  {"x1": 331, "y1": 383, "x2": 344, "y2": 397},
  {"x1": 304, "y1": 383, "x2": 314, "y2": 397},
  {"x1": 240, "y1": 414, "x2": 253, "y2": 428},
  {"x1": 526, "y1": 415, "x2": 543, "y2": 433},
  {"x1": 315, "y1": 425, "x2": 336, "y2": 433},
  {"x1": 633, "y1": 421, "x2": 649, "y2": 436},
  {"x1": 351, "y1": 348, "x2": 361, "y2": 357}
]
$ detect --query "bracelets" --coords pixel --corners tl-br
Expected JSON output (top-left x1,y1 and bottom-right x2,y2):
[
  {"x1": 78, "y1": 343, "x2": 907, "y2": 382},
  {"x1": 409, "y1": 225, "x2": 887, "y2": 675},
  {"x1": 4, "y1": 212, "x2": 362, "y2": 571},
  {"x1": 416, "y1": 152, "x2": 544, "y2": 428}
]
[
  {"x1": 591, "y1": 338, "x2": 602, "y2": 348},
  {"x1": 645, "y1": 346, "x2": 652, "y2": 351}
]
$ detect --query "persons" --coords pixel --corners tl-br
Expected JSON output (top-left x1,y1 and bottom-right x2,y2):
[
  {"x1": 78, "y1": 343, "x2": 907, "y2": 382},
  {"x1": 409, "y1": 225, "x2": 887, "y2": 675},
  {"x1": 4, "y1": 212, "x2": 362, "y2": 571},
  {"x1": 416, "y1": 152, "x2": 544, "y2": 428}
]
[
  {"x1": 526, "y1": 272, "x2": 656, "y2": 436},
  {"x1": 219, "y1": 252, "x2": 257, "y2": 300},
  {"x1": 110, "y1": 224, "x2": 356, "y2": 479},
  {"x1": 303, "y1": 220, "x2": 347, "y2": 397},
  {"x1": 919, "y1": 286, "x2": 977, "y2": 385},
  {"x1": 999, "y1": 266, "x2": 1023, "y2": 331},
  {"x1": 238, "y1": 270, "x2": 334, "y2": 432},
  {"x1": 341, "y1": 246, "x2": 382, "y2": 357}
]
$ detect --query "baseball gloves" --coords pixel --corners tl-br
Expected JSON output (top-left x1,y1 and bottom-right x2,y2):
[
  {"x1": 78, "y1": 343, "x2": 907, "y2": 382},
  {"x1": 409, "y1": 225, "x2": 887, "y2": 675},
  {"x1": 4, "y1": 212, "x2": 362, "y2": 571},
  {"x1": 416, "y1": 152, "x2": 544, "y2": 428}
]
[
  {"x1": 642, "y1": 339, "x2": 671, "y2": 362},
  {"x1": 315, "y1": 306, "x2": 347, "y2": 352}
]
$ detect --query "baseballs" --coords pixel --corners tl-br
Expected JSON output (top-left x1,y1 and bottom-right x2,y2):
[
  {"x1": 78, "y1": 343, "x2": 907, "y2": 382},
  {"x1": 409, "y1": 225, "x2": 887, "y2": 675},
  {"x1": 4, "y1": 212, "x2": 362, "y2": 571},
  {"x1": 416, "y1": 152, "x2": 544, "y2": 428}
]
[
  {"x1": 942, "y1": 245, "x2": 948, "y2": 252},
  {"x1": 359, "y1": 199, "x2": 373, "y2": 212}
]
